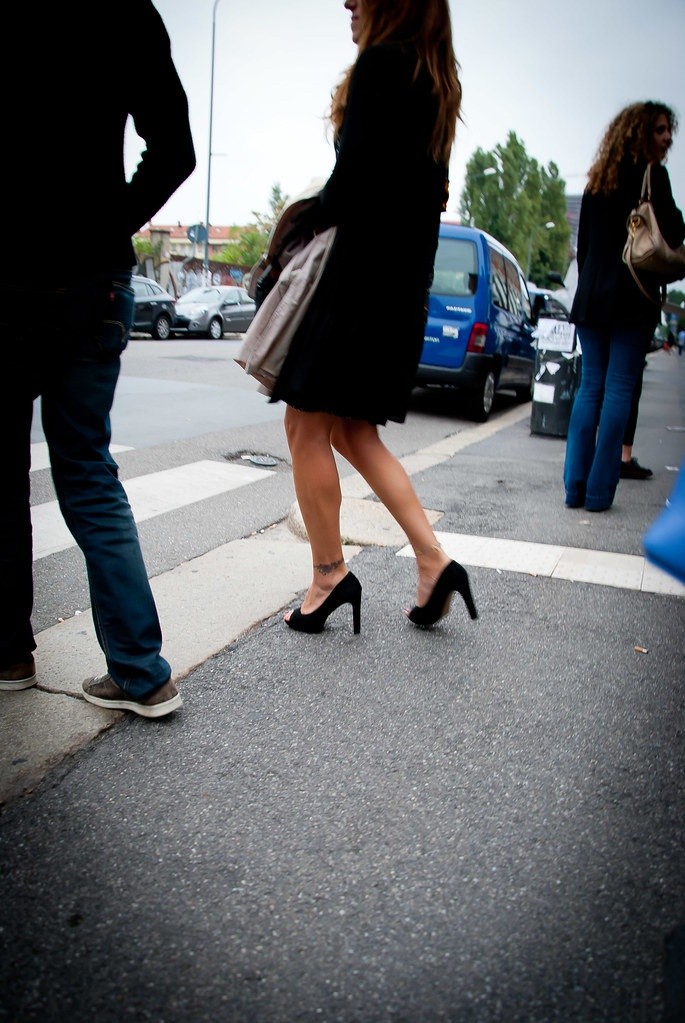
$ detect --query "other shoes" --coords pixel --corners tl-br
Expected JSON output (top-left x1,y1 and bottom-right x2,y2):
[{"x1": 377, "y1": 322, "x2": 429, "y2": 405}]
[{"x1": 619, "y1": 458, "x2": 653, "y2": 479}]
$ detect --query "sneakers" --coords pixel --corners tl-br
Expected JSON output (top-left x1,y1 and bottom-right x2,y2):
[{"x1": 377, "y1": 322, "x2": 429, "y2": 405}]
[
  {"x1": 82, "y1": 669, "x2": 181, "y2": 718},
  {"x1": 0, "y1": 661, "x2": 38, "y2": 691}
]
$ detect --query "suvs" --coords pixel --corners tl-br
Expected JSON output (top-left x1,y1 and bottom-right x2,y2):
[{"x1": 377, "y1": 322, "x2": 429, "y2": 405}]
[{"x1": 129, "y1": 274, "x2": 176, "y2": 340}]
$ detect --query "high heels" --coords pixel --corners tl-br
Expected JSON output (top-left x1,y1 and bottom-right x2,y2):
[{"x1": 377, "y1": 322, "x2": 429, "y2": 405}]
[
  {"x1": 284, "y1": 570, "x2": 362, "y2": 635},
  {"x1": 407, "y1": 560, "x2": 477, "y2": 626}
]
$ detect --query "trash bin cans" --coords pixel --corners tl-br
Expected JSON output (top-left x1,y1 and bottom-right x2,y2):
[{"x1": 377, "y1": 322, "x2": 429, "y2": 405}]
[{"x1": 530, "y1": 318, "x2": 583, "y2": 438}]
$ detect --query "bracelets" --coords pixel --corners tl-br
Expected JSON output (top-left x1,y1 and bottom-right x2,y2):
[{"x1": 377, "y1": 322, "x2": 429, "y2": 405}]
[{"x1": 255, "y1": 256, "x2": 269, "y2": 270}]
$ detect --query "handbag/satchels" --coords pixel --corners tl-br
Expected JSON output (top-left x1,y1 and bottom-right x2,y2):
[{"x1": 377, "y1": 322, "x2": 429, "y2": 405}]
[{"x1": 622, "y1": 163, "x2": 685, "y2": 304}]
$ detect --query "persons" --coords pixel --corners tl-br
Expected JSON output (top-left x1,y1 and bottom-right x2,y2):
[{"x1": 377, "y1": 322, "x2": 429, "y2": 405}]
[
  {"x1": 0, "y1": 0, "x2": 196, "y2": 719},
  {"x1": 246, "y1": 1, "x2": 478, "y2": 641},
  {"x1": 664, "y1": 330, "x2": 685, "y2": 355},
  {"x1": 565, "y1": 102, "x2": 685, "y2": 513}
]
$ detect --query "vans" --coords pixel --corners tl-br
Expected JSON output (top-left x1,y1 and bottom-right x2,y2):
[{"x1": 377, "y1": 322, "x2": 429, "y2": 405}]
[{"x1": 418, "y1": 222, "x2": 538, "y2": 421}]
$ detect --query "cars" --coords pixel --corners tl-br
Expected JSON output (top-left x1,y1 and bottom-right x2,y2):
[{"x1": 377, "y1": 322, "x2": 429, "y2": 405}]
[{"x1": 172, "y1": 285, "x2": 256, "y2": 339}]
